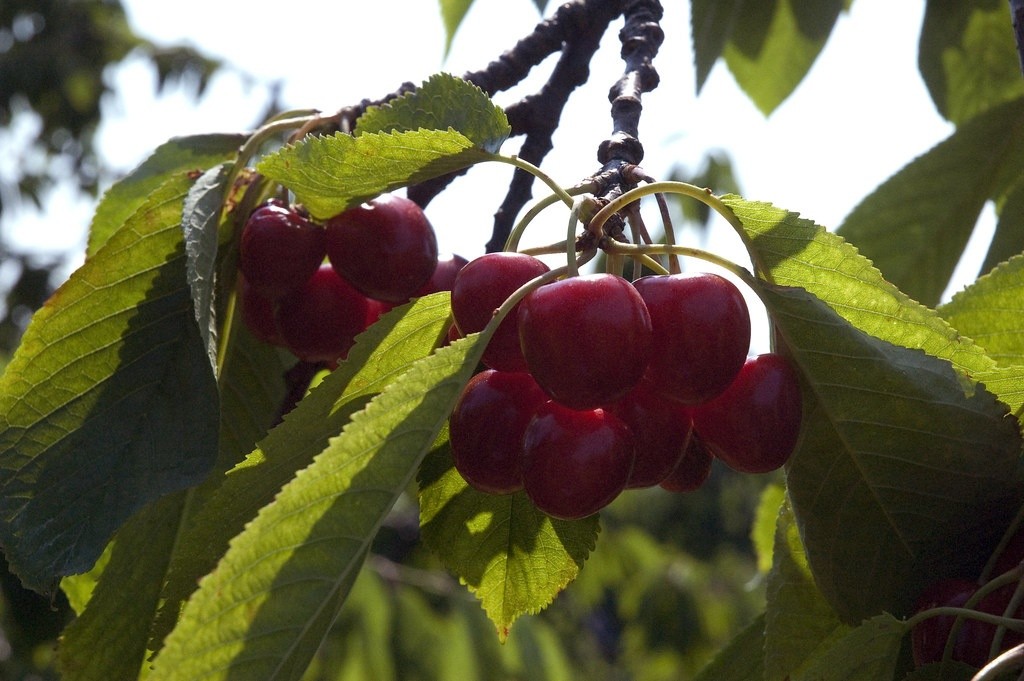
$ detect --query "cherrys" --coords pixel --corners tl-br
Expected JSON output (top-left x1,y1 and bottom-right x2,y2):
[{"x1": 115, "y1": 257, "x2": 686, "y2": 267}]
[
  {"x1": 906, "y1": 499, "x2": 1024, "y2": 681},
  {"x1": 231, "y1": 106, "x2": 805, "y2": 523}
]
